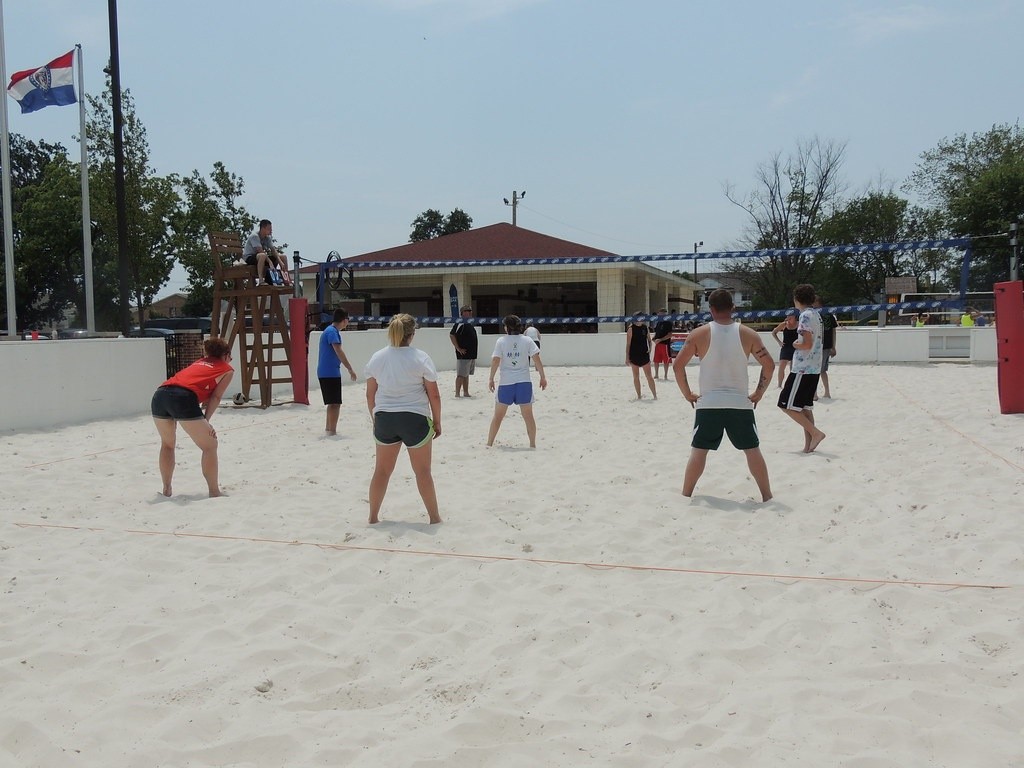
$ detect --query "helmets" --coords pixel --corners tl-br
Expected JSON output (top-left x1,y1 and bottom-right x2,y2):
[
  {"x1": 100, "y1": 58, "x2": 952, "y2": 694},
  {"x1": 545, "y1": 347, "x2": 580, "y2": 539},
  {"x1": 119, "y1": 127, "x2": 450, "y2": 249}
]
[
  {"x1": 785, "y1": 307, "x2": 799, "y2": 317},
  {"x1": 526, "y1": 319, "x2": 532, "y2": 325}
]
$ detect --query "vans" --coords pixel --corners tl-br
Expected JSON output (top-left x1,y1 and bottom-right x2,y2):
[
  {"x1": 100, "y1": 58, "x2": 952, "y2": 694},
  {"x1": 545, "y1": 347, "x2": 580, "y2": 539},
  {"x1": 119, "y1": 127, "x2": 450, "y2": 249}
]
[{"x1": 144, "y1": 316, "x2": 212, "y2": 331}]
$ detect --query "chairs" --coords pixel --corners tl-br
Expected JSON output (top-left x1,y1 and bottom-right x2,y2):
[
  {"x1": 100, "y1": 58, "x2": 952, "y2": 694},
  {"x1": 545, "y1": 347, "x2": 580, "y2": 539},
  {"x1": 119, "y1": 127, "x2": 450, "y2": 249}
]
[{"x1": 207, "y1": 228, "x2": 282, "y2": 291}]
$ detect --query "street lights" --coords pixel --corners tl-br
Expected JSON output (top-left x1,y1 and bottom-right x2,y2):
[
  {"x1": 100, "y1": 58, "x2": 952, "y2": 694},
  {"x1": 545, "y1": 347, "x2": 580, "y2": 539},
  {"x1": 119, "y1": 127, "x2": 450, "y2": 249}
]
[
  {"x1": 503, "y1": 191, "x2": 526, "y2": 226},
  {"x1": 694, "y1": 241, "x2": 704, "y2": 314}
]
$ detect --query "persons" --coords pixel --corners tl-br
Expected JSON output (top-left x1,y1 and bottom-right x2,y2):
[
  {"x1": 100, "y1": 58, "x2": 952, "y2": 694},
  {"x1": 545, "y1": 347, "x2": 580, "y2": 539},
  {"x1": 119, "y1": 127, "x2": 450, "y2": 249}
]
[
  {"x1": 487, "y1": 315, "x2": 547, "y2": 447},
  {"x1": 317, "y1": 307, "x2": 356, "y2": 437},
  {"x1": 812, "y1": 297, "x2": 838, "y2": 400},
  {"x1": 911, "y1": 310, "x2": 930, "y2": 326},
  {"x1": 652, "y1": 307, "x2": 674, "y2": 379},
  {"x1": 151, "y1": 338, "x2": 235, "y2": 497},
  {"x1": 242, "y1": 219, "x2": 286, "y2": 287},
  {"x1": 672, "y1": 289, "x2": 775, "y2": 503},
  {"x1": 524, "y1": 318, "x2": 541, "y2": 371},
  {"x1": 365, "y1": 313, "x2": 442, "y2": 525},
  {"x1": 649, "y1": 309, "x2": 695, "y2": 333},
  {"x1": 778, "y1": 284, "x2": 825, "y2": 453},
  {"x1": 772, "y1": 306, "x2": 799, "y2": 388},
  {"x1": 450, "y1": 306, "x2": 478, "y2": 398},
  {"x1": 626, "y1": 310, "x2": 658, "y2": 401},
  {"x1": 956, "y1": 305, "x2": 987, "y2": 327}
]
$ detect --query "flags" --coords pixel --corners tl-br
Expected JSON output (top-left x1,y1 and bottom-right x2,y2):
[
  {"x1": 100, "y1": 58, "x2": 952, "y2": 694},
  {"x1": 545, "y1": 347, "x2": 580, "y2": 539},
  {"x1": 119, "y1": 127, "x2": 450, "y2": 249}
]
[{"x1": 6, "y1": 50, "x2": 78, "y2": 114}]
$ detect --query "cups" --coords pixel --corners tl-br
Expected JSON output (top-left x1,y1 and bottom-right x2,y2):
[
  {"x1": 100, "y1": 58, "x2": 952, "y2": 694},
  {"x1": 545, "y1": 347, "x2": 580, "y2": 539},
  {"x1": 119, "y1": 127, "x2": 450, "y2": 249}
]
[
  {"x1": 32, "y1": 331, "x2": 38, "y2": 340},
  {"x1": 52, "y1": 331, "x2": 57, "y2": 340}
]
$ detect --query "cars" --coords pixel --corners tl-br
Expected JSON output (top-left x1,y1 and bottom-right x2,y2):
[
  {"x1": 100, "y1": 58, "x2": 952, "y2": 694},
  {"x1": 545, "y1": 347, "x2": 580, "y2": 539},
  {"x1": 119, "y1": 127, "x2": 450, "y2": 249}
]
[{"x1": 124, "y1": 328, "x2": 175, "y2": 338}]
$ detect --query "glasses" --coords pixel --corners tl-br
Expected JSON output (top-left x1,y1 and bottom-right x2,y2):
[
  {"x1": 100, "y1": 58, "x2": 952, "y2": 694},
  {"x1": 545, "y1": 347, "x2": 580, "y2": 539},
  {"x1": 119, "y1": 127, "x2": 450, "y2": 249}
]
[
  {"x1": 224, "y1": 352, "x2": 232, "y2": 361},
  {"x1": 343, "y1": 318, "x2": 349, "y2": 320},
  {"x1": 464, "y1": 310, "x2": 471, "y2": 312}
]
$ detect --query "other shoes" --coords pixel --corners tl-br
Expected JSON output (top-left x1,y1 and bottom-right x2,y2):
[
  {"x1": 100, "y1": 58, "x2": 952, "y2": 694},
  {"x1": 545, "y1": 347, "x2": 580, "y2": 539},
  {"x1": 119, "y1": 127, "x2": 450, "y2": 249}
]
[{"x1": 260, "y1": 282, "x2": 268, "y2": 286}]
